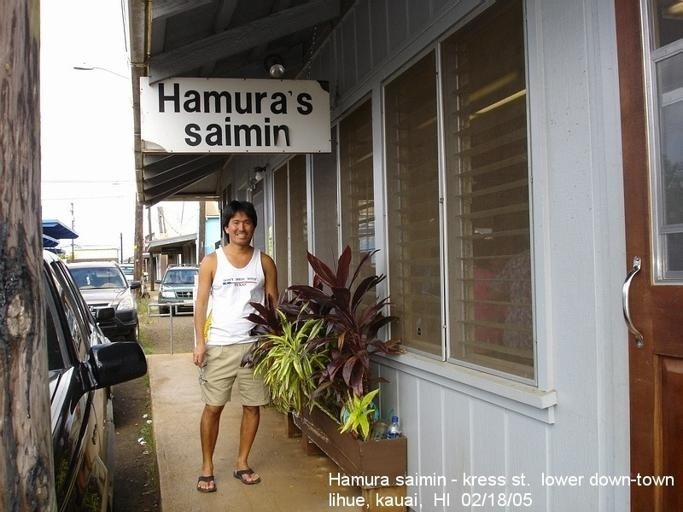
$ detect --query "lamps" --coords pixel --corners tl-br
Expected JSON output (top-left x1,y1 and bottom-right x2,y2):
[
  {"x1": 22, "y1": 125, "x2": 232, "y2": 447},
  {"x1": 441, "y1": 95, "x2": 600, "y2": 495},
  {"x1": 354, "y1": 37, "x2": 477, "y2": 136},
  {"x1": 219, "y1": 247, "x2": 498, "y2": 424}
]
[
  {"x1": 264, "y1": 53, "x2": 288, "y2": 79},
  {"x1": 255, "y1": 167, "x2": 266, "y2": 181}
]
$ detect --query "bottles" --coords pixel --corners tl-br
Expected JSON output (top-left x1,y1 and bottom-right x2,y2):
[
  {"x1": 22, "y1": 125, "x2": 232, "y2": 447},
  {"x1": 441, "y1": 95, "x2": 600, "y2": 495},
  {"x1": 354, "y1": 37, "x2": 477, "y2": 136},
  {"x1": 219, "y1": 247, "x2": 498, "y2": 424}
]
[{"x1": 387, "y1": 416, "x2": 402, "y2": 439}]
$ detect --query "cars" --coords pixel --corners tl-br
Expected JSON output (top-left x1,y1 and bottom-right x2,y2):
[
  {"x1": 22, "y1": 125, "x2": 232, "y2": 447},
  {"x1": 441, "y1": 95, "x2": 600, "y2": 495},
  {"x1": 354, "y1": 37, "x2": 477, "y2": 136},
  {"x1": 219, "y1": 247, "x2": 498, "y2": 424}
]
[
  {"x1": 41, "y1": 249, "x2": 150, "y2": 511},
  {"x1": 158, "y1": 264, "x2": 201, "y2": 314},
  {"x1": 64, "y1": 257, "x2": 141, "y2": 348}
]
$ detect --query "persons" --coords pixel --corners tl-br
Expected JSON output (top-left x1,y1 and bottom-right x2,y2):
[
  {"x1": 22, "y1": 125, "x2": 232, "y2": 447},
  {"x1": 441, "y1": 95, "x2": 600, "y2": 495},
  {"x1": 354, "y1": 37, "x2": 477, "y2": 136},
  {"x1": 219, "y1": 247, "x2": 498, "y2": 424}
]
[
  {"x1": 191, "y1": 199, "x2": 278, "y2": 493},
  {"x1": 405, "y1": 218, "x2": 536, "y2": 363}
]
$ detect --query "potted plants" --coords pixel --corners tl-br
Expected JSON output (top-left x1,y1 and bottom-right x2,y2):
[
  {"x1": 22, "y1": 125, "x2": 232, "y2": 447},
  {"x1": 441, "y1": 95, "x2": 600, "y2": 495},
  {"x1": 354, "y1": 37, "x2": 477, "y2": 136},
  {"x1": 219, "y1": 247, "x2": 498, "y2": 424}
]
[{"x1": 240, "y1": 247, "x2": 408, "y2": 512}]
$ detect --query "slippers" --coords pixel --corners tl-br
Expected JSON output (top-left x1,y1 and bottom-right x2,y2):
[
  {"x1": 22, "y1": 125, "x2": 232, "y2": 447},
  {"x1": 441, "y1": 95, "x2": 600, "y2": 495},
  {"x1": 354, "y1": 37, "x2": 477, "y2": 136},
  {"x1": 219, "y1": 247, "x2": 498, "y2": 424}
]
[
  {"x1": 234, "y1": 467, "x2": 260, "y2": 484},
  {"x1": 196, "y1": 475, "x2": 216, "y2": 492}
]
